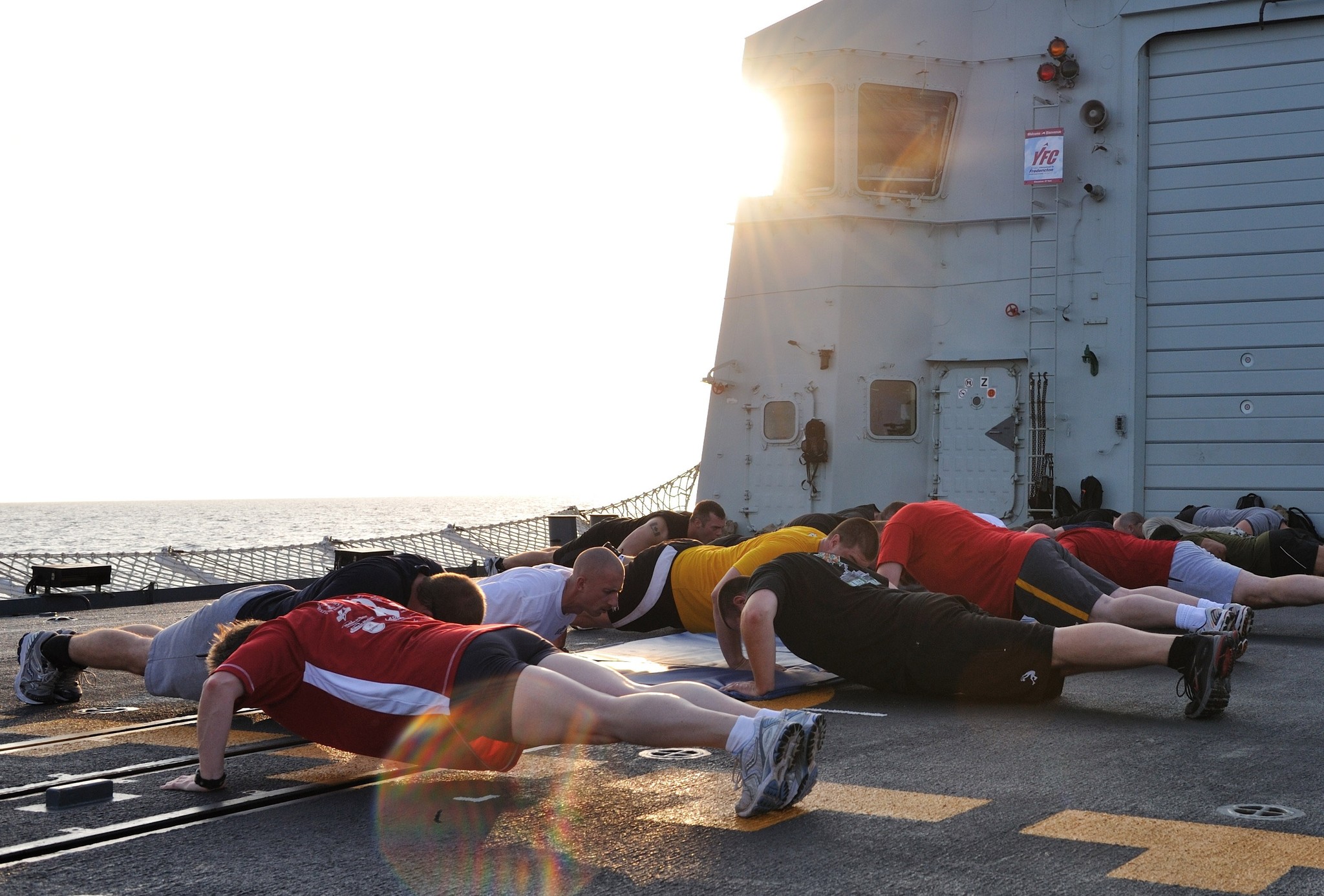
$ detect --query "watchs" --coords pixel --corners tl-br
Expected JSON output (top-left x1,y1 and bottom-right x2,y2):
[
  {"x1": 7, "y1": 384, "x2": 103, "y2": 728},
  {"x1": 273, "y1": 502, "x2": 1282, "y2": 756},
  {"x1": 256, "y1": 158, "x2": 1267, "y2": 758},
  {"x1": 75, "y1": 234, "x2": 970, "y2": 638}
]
[{"x1": 194, "y1": 768, "x2": 227, "y2": 790}]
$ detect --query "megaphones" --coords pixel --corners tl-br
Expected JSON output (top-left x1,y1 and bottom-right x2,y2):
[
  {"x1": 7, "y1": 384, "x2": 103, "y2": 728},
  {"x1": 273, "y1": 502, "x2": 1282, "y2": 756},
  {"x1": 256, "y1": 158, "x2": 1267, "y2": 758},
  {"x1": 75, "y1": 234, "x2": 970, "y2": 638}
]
[{"x1": 1078, "y1": 99, "x2": 1111, "y2": 132}]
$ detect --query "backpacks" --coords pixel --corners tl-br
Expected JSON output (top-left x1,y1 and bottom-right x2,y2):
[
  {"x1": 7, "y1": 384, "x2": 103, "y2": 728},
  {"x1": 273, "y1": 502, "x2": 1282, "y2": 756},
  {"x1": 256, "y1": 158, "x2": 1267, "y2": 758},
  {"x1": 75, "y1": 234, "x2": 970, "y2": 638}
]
[
  {"x1": 1028, "y1": 486, "x2": 1081, "y2": 520},
  {"x1": 1271, "y1": 504, "x2": 1319, "y2": 541},
  {"x1": 1081, "y1": 476, "x2": 1104, "y2": 511},
  {"x1": 1236, "y1": 493, "x2": 1266, "y2": 510}
]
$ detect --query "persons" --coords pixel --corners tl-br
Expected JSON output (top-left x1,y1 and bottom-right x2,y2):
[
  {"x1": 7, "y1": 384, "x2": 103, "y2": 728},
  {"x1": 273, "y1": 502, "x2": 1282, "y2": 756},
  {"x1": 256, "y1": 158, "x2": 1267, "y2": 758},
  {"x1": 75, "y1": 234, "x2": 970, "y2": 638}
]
[
  {"x1": 836, "y1": 501, "x2": 909, "y2": 520},
  {"x1": 488, "y1": 500, "x2": 726, "y2": 576},
  {"x1": 704, "y1": 511, "x2": 848, "y2": 548},
  {"x1": 1024, "y1": 523, "x2": 1324, "y2": 609},
  {"x1": 160, "y1": 592, "x2": 827, "y2": 818},
  {"x1": 574, "y1": 516, "x2": 881, "y2": 633},
  {"x1": 1175, "y1": 505, "x2": 1291, "y2": 537},
  {"x1": 875, "y1": 502, "x2": 1255, "y2": 659},
  {"x1": 1148, "y1": 524, "x2": 1324, "y2": 576},
  {"x1": 1114, "y1": 511, "x2": 1254, "y2": 540},
  {"x1": 477, "y1": 546, "x2": 625, "y2": 650},
  {"x1": 18, "y1": 553, "x2": 486, "y2": 705},
  {"x1": 719, "y1": 553, "x2": 1239, "y2": 719}
]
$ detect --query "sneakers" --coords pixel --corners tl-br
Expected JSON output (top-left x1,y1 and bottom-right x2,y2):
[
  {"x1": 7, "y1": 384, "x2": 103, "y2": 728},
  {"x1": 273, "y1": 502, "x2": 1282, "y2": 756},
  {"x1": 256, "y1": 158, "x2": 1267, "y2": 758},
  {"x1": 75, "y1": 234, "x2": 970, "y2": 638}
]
[
  {"x1": 1223, "y1": 603, "x2": 1254, "y2": 659},
  {"x1": 51, "y1": 628, "x2": 95, "y2": 704},
  {"x1": 484, "y1": 556, "x2": 506, "y2": 576},
  {"x1": 731, "y1": 715, "x2": 805, "y2": 818},
  {"x1": 1188, "y1": 607, "x2": 1236, "y2": 634},
  {"x1": 603, "y1": 541, "x2": 624, "y2": 557},
  {"x1": 778, "y1": 708, "x2": 827, "y2": 808},
  {"x1": 14, "y1": 629, "x2": 71, "y2": 705},
  {"x1": 1176, "y1": 631, "x2": 1240, "y2": 719}
]
